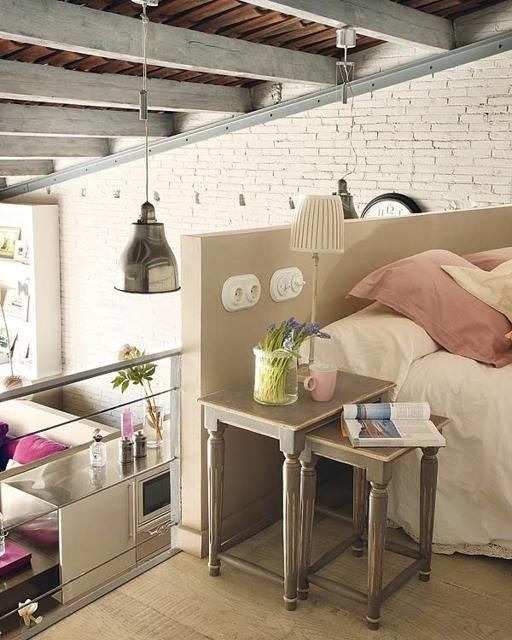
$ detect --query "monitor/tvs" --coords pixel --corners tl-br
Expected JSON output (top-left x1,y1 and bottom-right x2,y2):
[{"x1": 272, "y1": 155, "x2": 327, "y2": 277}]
[{"x1": 142, "y1": 470, "x2": 171, "y2": 516}]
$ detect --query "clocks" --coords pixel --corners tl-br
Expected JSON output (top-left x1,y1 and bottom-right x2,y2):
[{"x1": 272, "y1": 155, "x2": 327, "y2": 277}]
[{"x1": 360, "y1": 193, "x2": 422, "y2": 218}]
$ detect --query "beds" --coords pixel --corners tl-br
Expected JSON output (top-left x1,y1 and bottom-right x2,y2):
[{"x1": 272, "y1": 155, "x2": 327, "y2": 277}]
[{"x1": 293, "y1": 347, "x2": 512, "y2": 560}]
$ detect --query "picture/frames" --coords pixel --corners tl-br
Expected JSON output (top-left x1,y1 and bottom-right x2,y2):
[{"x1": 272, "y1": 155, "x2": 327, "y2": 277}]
[{"x1": 0, "y1": 226, "x2": 20, "y2": 259}]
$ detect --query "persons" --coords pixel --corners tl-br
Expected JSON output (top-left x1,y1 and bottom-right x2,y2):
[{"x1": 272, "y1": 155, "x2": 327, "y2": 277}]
[{"x1": 2, "y1": 375, "x2": 25, "y2": 398}]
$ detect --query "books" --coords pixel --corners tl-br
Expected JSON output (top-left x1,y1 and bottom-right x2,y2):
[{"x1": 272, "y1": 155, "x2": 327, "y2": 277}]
[
  {"x1": 339, "y1": 399, "x2": 449, "y2": 449},
  {"x1": 0, "y1": 225, "x2": 34, "y2": 377}
]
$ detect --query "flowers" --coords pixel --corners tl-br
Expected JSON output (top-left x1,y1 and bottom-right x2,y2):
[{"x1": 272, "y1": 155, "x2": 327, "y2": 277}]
[
  {"x1": 111, "y1": 344, "x2": 165, "y2": 442},
  {"x1": 259, "y1": 317, "x2": 330, "y2": 403}
]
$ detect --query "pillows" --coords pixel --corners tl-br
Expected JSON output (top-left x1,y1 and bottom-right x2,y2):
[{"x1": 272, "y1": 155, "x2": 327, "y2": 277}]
[
  {"x1": 0, "y1": 420, "x2": 72, "y2": 490},
  {"x1": 319, "y1": 247, "x2": 512, "y2": 400}
]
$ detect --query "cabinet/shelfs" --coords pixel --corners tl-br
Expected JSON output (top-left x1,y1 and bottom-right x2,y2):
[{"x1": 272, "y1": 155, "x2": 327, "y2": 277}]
[{"x1": 0, "y1": 202, "x2": 63, "y2": 410}]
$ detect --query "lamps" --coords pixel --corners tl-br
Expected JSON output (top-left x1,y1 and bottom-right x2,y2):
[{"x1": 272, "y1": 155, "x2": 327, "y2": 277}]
[
  {"x1": 330, "y1": 28, "x2": 360, "y2": 219},
  {"x1": 111, "y1": 0, "x2": 182, "y2": 294},
  {"x1": 289, "y1": 193, "x2": 345, "y2": 385}
]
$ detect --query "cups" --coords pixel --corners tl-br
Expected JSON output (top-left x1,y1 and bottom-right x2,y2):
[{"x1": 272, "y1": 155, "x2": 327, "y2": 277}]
[{"x1": 303, "y1": 363, "x2": 337, "y2": 401}]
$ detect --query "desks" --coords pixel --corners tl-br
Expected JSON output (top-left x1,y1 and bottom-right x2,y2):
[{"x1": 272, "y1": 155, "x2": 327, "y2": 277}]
[{"x1": 0, "y1": 541, "x2": 59, "y2": 635}]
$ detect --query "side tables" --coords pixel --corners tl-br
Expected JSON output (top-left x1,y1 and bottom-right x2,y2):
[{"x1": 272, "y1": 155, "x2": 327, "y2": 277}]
[
  {"x1": 296, "y1": 414, "x2": 450, "y2": 630},
  {"x1": 198, "y1": 363, "x2": 396, "y2": 612}
]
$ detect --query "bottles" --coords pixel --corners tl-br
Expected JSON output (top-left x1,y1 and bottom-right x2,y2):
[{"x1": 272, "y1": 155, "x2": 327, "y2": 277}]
[
  {"x1": 134, "y1": 431, "x2": 147, "y2": 458},
  {"x1": 90, "y1": 435, "x2": 106, "y2": 467},
  {"x1": 119, "y1": 436, "x2": 134, "y2": 463},
  {"x1": 121, "y1": 407, "x2": 133, "y2": 443}
]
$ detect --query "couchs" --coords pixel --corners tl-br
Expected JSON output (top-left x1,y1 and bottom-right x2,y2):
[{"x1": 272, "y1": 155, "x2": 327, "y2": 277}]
[{"x1": 0, "y1": 398, "x2": 128, "y2": 552}]
